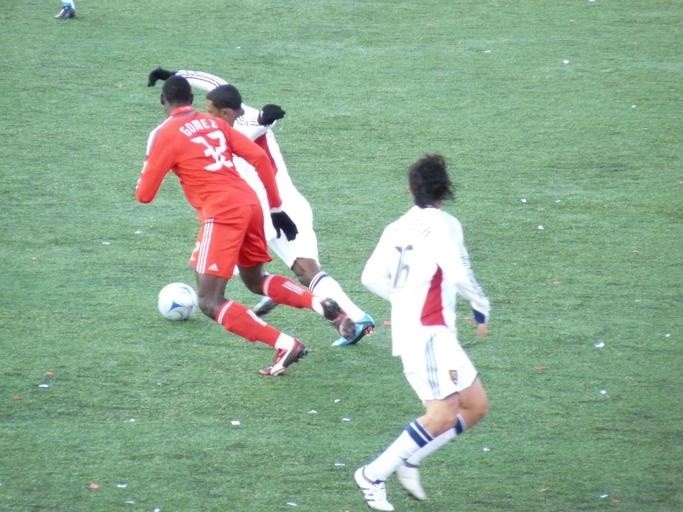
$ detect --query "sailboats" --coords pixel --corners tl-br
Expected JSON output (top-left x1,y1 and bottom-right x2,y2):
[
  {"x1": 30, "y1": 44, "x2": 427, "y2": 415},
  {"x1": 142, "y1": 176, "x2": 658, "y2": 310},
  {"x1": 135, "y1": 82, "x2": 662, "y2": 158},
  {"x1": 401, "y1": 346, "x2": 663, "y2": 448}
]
[{"x1": 157, "y1": 283, "x2": 197, "y2": 321}]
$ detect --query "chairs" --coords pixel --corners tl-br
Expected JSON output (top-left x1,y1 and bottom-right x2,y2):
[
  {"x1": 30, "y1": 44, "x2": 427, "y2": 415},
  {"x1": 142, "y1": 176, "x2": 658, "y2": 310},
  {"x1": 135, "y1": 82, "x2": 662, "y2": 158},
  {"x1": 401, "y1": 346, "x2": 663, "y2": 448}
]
[
  {"x1": 317, "y1": 297, "x2": 374, "y2": 346},
  {"x1": 353, "y1": 468, "x2": 394, "y2": 511},
  {"x1": 260, "y1": 338, "x2": 306, "y2": 376},
  {"x1": 54, "y1": 6, "x2": 75, "y2": 18},
  {"x1": 396, "y1": 457, "x2": 427, "y2": 500}
]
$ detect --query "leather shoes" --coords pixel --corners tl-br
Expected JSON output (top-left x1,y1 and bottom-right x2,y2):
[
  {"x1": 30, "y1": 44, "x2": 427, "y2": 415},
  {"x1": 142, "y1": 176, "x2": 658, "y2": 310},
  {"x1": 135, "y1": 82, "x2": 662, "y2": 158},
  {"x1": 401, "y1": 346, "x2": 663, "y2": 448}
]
[
  {"x1": 258, "y1": 104, "x2": 285, "y2": 125},
  {"x1": 271, "y1": 211, "x2": 297, "y2": 241},
  {"x1": 148, "y1": 68, "x2": 176, "y2": 86}
]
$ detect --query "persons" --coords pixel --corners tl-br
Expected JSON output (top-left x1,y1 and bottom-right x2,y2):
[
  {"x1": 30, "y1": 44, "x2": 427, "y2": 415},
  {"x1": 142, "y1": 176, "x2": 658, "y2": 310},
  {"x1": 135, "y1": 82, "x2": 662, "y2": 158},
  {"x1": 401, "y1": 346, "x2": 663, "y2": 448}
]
[
  {"x1": 54, "y1": 0, "x2": 78, "y2": 18},
  {"x1": 351, "y1": 151, "x2": 493, "y2": 511},
  {"x1": 135, "y1": 74, "x2": 359, "y2": 376},
  {"x1": 147, "y1": 68, "x2": 376, "y2": 348}
]
